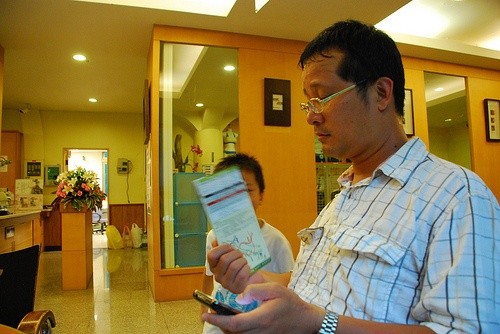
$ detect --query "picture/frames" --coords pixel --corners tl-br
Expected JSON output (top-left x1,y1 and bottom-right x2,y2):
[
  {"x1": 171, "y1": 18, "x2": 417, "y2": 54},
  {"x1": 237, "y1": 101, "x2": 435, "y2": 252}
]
[
  {"x1": 264, "y1": 78, "x2": 291, "y2": 127},
  {"x1": 400, "y1": 89, "x2": 415, "y2": 137},
  {"x1": 484, "y1": 99, "x2": 500, "y2": 142}
]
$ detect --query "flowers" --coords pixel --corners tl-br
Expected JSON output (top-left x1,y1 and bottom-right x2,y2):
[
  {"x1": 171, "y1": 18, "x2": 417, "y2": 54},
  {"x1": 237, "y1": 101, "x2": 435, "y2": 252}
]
[{"x1": 50, "y1": 166, "x2": 108, "y2": 213}]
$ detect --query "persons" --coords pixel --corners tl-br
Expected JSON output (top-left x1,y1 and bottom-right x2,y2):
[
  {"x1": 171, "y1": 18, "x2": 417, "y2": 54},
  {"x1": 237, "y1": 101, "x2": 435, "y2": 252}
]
[
  {"x1": 199, "y1": 153, "x2": 296, "y2": 334},
  {"x1": 222, "y1": 128, "x2": 238, "y2": 156},
  {"x1": 200, "y1": 20, "x2": 500, "y2": 334}
]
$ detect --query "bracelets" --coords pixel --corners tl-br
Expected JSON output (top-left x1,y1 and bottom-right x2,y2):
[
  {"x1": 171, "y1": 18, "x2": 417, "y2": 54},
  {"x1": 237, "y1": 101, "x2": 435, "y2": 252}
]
[{"x1": 318, "y1": 310, "x2": 340, "y2": 334}]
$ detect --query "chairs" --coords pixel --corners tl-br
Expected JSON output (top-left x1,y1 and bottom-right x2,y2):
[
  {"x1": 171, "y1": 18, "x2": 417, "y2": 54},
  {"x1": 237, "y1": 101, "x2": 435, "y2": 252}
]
[
  {"x1": 0, "y1": 244, "x2": 56, "y2": 334},
  {"x1": 92, "y1": 206, "x2": 107, "y2": 234}
]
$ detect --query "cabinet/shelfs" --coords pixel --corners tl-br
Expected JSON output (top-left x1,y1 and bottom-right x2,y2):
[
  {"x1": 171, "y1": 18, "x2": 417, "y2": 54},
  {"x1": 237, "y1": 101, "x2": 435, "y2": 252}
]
[
  {"x1": 0, "y1": 216, "x2": 47, "y2": 257},
  {"x1": 0, "y1": 130, "x2": 23, "y2": 194}
]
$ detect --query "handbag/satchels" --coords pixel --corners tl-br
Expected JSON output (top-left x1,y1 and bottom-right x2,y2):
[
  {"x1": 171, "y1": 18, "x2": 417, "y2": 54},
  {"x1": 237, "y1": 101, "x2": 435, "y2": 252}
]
[
  {"x1": 106, "y1": 225, "x2": 122, "y2": 250},
  {"x1": 122, "y1": 226, "x2": 133, "y2": 250},
  {"x1": 131, "y1": 223, "x2": 143, "y2": 248}
]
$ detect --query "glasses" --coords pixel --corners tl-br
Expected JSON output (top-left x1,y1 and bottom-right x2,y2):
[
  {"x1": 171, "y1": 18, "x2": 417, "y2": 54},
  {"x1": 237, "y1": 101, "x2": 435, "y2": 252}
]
[{"x1": 300, "y1": 79, "x2": 368, "y2": 114}]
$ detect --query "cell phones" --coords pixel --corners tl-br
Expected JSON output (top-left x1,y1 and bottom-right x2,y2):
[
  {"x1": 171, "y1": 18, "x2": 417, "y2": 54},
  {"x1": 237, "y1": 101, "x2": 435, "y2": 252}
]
[{"x1": 192, "y1": 289, "x2": 242, "y2": 316}]
